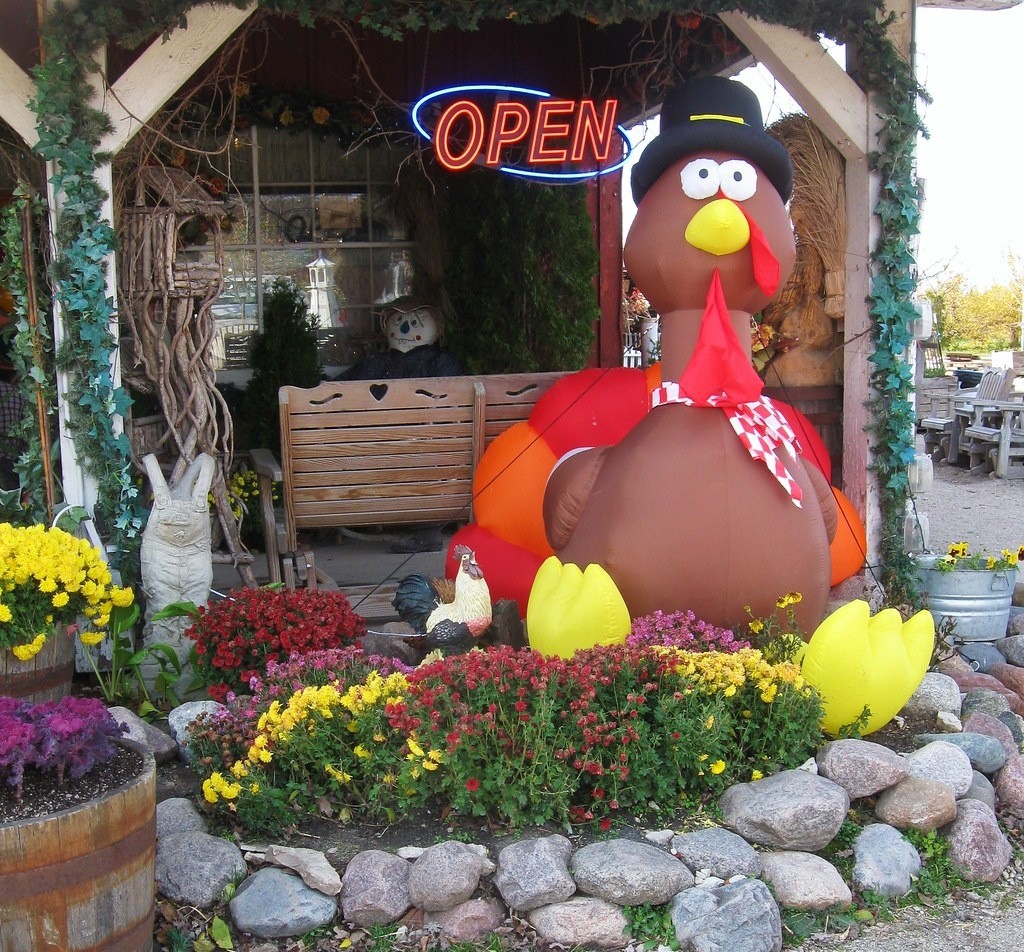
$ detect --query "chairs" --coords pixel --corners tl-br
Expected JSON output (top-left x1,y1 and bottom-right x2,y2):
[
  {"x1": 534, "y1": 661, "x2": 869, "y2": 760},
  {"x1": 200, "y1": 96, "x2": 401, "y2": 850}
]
[
  {"x1": 964, "y1": 394, "x2": 1024, "y2": 481},
  {"x1": 920, "y1": 366, "x2": 1018, "y2": 463}
]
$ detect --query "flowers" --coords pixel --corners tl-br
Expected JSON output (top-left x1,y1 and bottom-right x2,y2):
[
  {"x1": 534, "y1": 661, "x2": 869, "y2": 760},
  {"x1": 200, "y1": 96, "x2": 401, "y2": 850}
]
[
  {"x1": 173, "y1": 578, "x2": 831, "y2": 831},
  {"x1": 0, "y1": 521, "x2": 137, "y2": 661},
  {"x1": 935, "y1": 539, "x2": 1024, "y2": 585},
  {"x1": 136, "y1": 467, "x2": 281, "y2": 523}
]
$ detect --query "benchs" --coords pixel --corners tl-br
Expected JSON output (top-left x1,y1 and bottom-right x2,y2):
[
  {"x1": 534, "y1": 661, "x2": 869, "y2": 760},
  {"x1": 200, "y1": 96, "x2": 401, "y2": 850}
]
[{"x1": 246, "y1": 369, "x2": 586, "y2": 624}]
